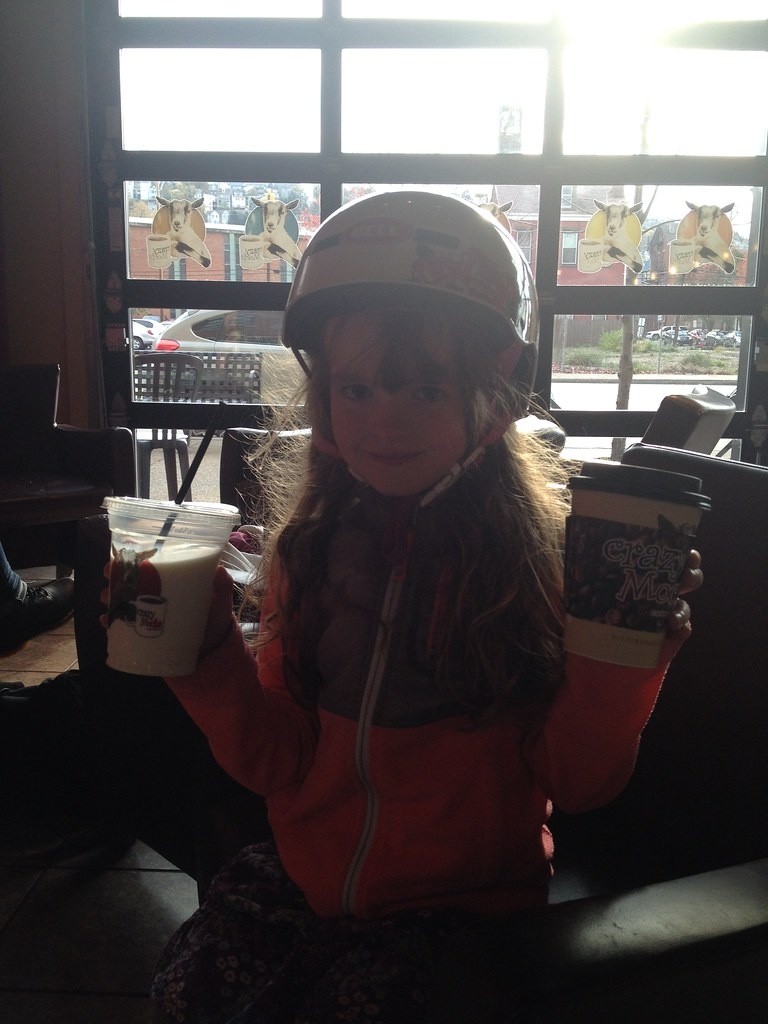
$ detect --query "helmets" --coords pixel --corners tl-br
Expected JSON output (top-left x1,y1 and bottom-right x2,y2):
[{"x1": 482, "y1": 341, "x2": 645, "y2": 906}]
[{"x1": 280, "y1": 192, "x2": 531, "y2": 350}]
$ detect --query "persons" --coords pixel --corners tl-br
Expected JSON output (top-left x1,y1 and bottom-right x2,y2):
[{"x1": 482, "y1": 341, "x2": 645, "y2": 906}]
[
  {"x1": 0, "y1": 541, "x2": 78, "y2": 658},
  {"x1": 97, "y1": 190, "x2": 705, "y2": 1023}
]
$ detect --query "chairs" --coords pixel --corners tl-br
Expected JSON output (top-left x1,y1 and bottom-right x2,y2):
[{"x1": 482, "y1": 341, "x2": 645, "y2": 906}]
[
  {"x1": 0, "y1": 363, "x2": 134, "y2": 580},
  {"x1": 134, "y1": 352, "x2": 204, "y2": 505},
  {"x1": 516, "y1": 441, "x2": 768, "y2": 1024}
]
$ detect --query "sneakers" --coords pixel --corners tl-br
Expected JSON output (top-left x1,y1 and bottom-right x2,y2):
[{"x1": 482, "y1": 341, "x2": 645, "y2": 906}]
[{"x1": 0, "y1": 578, "x2": 74, "y2": 659}]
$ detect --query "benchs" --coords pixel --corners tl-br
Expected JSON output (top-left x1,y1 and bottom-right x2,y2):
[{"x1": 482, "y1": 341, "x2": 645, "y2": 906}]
[{"x1": 133, "y1": 349, "x2": 264, "y2": 436}]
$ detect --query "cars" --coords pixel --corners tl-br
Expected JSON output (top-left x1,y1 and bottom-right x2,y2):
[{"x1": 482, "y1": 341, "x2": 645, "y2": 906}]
[
  {"x1": 154, "y1": 310, "x2": 305, "y2": 405},
  {"x1": 646, "y1": 326, "x2": 741, "y2": 348},
  {"x1": 132, "y1": 315, "x2": 174, "y2": 350}
]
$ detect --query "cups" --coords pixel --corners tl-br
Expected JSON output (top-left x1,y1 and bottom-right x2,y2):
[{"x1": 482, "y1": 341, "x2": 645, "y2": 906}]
[
  {"x1": 103, "y1": 495, "x2": 242, "y2": 679},
  {"x1": 563, "y1": 462, "x2": 712, "y2": 670}
]
